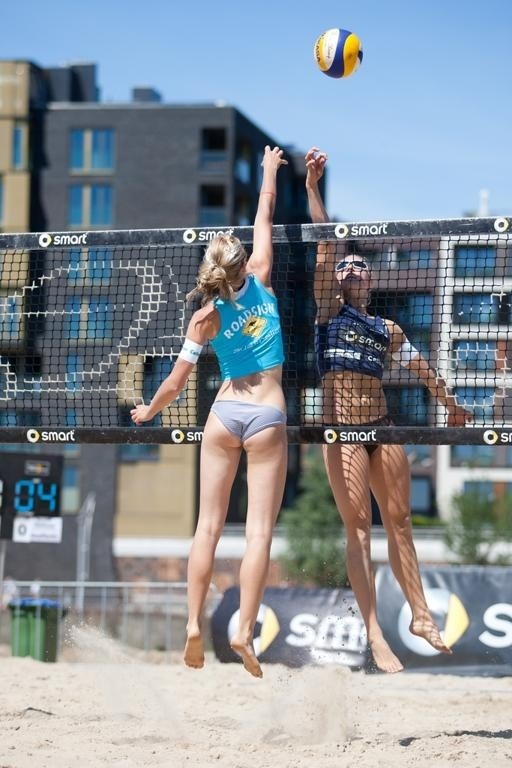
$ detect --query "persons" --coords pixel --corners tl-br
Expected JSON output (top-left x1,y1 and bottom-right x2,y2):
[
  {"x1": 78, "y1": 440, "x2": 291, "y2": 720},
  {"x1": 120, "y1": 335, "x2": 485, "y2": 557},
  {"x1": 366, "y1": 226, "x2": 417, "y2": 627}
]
[
  {"x1": 128, "y1": 142, "x2": 291, "y2": 679},
  {"x1": 304, "y1": 148, "x2": 476, "y2": 675}
]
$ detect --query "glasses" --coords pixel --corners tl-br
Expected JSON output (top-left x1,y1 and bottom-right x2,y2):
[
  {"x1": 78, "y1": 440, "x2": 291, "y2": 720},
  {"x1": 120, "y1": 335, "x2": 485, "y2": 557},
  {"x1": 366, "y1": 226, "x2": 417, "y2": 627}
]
[{"x1": 336, "y1": 260, "x2": 368, "y2": 272}]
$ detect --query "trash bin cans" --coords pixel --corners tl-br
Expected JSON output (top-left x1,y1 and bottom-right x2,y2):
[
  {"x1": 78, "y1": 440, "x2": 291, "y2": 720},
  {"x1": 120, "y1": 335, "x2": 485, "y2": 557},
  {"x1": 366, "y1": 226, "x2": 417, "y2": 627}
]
[{"x1": 9, "y1": 598, "x2": 62, "y2": 662}]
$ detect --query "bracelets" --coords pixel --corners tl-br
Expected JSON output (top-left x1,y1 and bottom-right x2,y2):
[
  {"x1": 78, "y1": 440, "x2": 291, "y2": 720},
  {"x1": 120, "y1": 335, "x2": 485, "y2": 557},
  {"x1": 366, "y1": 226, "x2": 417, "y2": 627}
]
[{"x1": 259, "y1": 189, "x2": 278, "y2": 199}]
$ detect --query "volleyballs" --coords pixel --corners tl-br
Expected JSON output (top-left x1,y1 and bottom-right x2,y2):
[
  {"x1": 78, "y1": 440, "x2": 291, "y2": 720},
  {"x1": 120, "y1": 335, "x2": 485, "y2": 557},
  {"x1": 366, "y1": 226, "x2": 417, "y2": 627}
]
[{"x1": 314, "y1": 28, "x2": 362, "y2": 79}]
